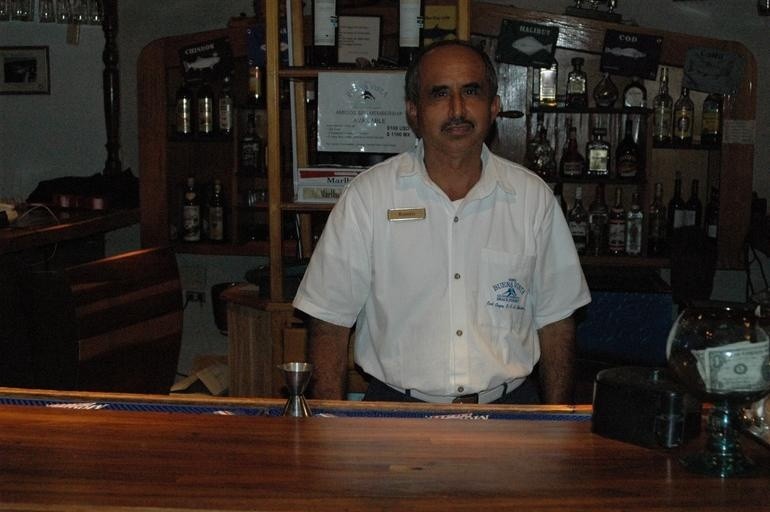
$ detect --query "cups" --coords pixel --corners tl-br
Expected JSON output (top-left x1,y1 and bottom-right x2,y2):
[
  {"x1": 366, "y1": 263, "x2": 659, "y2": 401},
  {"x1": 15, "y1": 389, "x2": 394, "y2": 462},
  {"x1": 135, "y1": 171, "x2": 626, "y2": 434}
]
[{"x1": 0, "y1": 1, "x2": 108, "y2": 26}]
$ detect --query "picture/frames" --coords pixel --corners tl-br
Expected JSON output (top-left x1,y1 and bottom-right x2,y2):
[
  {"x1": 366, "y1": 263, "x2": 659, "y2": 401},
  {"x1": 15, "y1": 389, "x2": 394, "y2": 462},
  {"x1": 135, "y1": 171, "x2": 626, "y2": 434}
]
[{"x1": 0, "y1": 45, "x2": 50, "y2": 94}]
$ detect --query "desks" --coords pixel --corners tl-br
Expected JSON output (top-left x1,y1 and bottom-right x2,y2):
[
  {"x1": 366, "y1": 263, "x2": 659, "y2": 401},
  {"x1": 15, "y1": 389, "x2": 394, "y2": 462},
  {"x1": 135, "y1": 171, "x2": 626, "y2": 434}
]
[{"x1": 0, "y1": 207, "x2": 140, "y2": 257}]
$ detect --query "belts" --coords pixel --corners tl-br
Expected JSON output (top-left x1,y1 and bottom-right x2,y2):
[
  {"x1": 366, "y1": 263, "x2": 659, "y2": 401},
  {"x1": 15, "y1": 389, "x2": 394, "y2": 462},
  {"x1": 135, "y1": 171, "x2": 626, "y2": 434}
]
[{"x1": 378, "y1": 371, "x2": 534, "y2": 414}]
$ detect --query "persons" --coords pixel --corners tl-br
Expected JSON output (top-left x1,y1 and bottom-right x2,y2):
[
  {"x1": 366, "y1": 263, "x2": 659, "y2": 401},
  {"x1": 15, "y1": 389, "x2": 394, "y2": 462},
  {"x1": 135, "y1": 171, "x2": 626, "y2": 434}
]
[{"x1": 291, "y1": 40, "x2": 592, "y2": 404}]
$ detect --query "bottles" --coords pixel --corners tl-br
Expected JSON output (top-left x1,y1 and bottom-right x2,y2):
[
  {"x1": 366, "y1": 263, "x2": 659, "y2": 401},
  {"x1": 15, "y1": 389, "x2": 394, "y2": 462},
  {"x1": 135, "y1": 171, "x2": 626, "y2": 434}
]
[
  {"x1": 175, "y1": 60, "x2": 266, "y2": 243},
  {"x1": 532, "y1": 49, "x2": 724, "y2": 258},
  {"x1": 311, "y1": 0, "x2": 340, "y2": 66},
  {"x1": 397, "y1": 0, "x2": 420, "y2": 68}
]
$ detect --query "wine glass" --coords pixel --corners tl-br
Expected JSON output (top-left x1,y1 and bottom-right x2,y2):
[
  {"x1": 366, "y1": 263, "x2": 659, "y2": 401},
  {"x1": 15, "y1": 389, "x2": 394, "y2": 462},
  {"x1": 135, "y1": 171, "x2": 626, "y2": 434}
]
[
  {"x1": 276, "y1": 360, "x2": 318, "y2": 416},
  {"x1": 663, "y1": 305, "x2": 770, "y2": 479}
]
